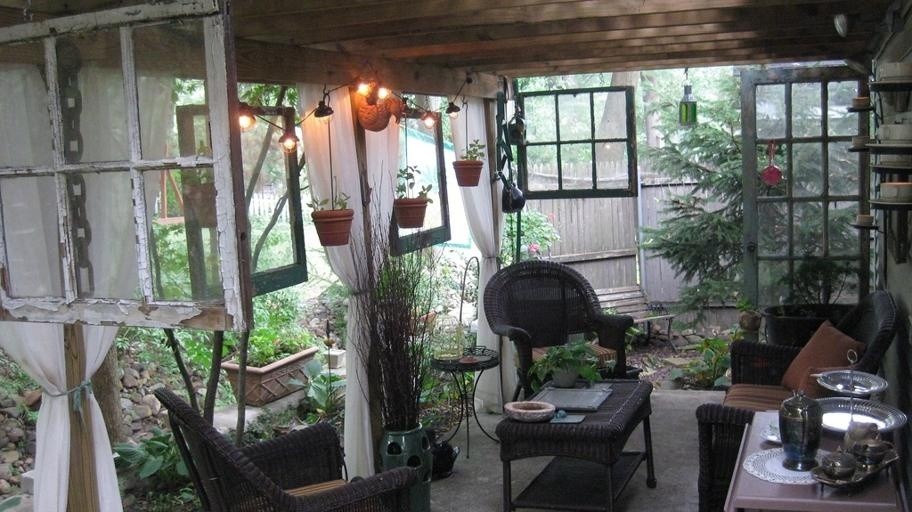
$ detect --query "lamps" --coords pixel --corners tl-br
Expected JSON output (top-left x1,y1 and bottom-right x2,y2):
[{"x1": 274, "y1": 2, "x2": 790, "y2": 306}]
[
  {"x1": 357, "y1": 82, "x2": 370, "y2": 95},
  {"x1": 378, "y1": 88, "x2": 390, "y2": 99},
  {"x1": 314, "y1": 98, "x2": 334, "y2": 120},
  {"x1": 447, "y1": 102, "x2": 461, "y2": 118},
  {"x1": 422, "y1": 111, "x2": 436, "y2": 128},
  {"x1": 238, "y1": 103, "x2": 256, "y2": 131},
  {"x1": 277, "y1": 131, "x2": 299, "y2": 153}
]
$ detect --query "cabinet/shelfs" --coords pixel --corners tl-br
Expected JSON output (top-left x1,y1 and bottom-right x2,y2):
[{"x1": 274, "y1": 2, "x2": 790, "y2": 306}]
[{"x1": 846, "y1": 62, "x2": 912, "y2": 228}]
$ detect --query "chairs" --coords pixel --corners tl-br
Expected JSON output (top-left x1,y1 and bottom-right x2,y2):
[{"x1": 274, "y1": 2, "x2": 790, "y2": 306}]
[
  {"x1": 155, "y1": 388, "x2": 431, "y2": 510},
  {"x1": 695, "y1": 289, "x2": 895, "y2": 511},
  {"x1": 484, "y1": 261, "x2": 642, "y2": 397}
]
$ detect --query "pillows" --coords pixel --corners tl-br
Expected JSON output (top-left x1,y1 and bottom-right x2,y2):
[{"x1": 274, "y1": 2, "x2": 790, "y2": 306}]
[{"x1": 781, "y1": 321, "x2": 869, "y2": 400}]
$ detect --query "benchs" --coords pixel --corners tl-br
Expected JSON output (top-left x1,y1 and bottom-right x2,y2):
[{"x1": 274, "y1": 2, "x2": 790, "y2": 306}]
[{"x1": 592, "y1": 285, "x2": 678, "y2": 354}]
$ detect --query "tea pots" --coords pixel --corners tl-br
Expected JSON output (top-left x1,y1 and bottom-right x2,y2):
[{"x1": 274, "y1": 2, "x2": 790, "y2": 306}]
[{"x1": 778, "y1": 389, "x2": 823, "y2": 471}]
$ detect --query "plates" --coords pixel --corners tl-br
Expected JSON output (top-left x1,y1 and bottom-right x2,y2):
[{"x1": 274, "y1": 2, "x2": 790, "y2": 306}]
[{"x1": 760, "y1": 428, "x2": 780, "y2": 448}]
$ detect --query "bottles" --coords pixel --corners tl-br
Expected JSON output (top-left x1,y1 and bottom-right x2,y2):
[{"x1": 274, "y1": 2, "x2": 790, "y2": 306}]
[{"x1": 678, "y1": 83, "x2": 697, "y2": 126}]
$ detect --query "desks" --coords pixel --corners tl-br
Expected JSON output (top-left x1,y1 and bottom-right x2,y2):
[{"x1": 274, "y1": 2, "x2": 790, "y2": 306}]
[
  {"x1": 722, "y1": 410, "x2": 907, "y2": 512},
  {"x1": 495, "y1": 378, "x2": 657, "y2": 511},
  {"x1": 429, "y1": 348, "x2": 500, "y2": 459}
]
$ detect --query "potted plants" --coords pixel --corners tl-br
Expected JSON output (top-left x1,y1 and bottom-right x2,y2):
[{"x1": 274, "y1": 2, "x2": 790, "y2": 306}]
[
  {"x1": 528, "y1": 340, "x2": 601, "y2": 392},
  {"x1": 307, "y1": 178, "x2": 352, "y2": 247},
  {"x1": 453, "y1": 138, "x2": 485, "y2": 186},
  {"x1": 761, "y1": 250, "x2": 856, "y2": 347},
  {"x1": 394, "y1": 164, "x2": 433, "y2": 228},
  {"x1": 218, "y1": 326, "x2": 320, "y2": 406},
  {"x1": 338, "y1": 160, "x2": 438, "y2": 485},
  {"x1": 181, "y1": 139, "x2": 220, "y2": 229}
]
